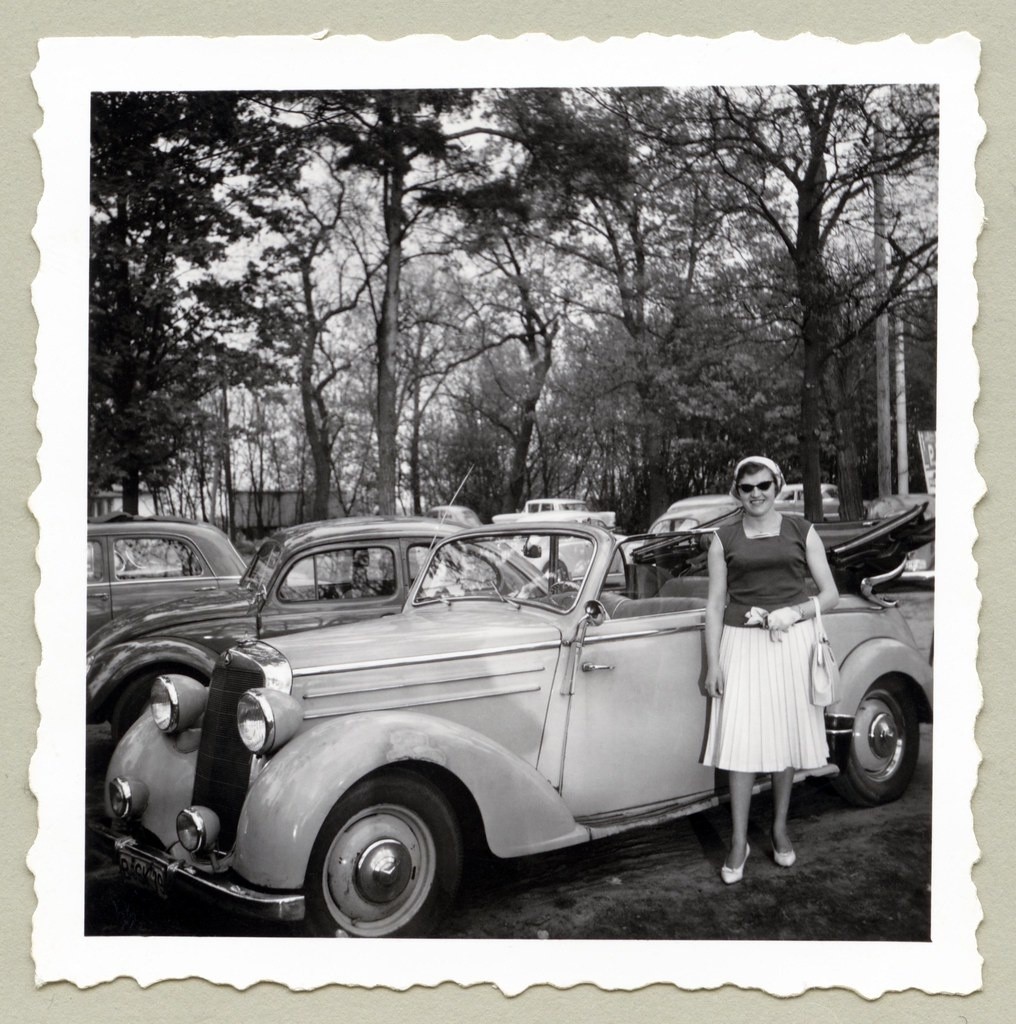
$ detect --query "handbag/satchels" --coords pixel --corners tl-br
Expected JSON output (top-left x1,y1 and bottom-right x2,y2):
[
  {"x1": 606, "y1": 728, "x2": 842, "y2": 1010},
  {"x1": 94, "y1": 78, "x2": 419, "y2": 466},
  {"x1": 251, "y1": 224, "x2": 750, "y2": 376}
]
[{"x1": 810, "y1": 641, "x2": 843, "y2": 707}]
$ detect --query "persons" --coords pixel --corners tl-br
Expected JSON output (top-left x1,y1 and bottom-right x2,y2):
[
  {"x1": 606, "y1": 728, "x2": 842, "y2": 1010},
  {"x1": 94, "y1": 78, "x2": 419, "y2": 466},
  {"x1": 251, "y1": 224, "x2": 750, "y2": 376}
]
[{"x1": 704, "y1": 456, "x2": 839, "y2": 883}]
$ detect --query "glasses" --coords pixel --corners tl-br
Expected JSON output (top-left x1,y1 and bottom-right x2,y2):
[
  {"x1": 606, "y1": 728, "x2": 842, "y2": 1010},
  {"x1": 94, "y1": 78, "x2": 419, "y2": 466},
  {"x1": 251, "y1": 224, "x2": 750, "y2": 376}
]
[{"x1": 736, "y1": 481, "x2": 774, "y2": 492}]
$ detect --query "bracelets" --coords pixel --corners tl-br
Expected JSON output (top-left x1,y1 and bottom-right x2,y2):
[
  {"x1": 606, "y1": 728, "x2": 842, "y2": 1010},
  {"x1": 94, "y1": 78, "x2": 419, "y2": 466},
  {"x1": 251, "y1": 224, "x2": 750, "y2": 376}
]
[{"x1": 798, "y1": 605, "x2": 805, "y2": 618}]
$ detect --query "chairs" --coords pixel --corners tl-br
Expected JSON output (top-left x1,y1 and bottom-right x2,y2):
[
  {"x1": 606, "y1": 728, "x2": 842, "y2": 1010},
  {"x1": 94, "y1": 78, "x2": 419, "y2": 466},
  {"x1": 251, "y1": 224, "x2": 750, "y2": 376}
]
[{"x1": 540, "y1": 577, "x2": 715, "y2": 618}]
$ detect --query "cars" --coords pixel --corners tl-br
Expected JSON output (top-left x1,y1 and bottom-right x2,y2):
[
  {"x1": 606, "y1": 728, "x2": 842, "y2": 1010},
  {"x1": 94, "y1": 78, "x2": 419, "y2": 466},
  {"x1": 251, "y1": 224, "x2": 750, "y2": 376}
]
[{"x1": 86, "y1": 485, "x2": 939, "y2": 939}]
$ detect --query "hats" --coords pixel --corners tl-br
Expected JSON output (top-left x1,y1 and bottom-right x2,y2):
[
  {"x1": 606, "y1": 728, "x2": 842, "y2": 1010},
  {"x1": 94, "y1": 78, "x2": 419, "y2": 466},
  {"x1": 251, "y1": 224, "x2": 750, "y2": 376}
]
[{"x1": 729, "y1": 456, "x2": 785, "y2": 502}]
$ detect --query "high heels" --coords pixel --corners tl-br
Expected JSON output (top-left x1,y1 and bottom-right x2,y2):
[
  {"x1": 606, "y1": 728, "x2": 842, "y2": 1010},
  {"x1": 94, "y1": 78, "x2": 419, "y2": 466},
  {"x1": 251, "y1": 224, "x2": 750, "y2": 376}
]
[
  {"x1": 721, "y1": 842, "x2": 751, "y2": 885},
  {"x1": 768, "y1": 829, "x2": 796, "y2": 867}
]
